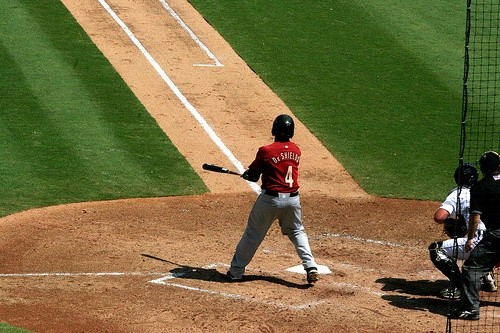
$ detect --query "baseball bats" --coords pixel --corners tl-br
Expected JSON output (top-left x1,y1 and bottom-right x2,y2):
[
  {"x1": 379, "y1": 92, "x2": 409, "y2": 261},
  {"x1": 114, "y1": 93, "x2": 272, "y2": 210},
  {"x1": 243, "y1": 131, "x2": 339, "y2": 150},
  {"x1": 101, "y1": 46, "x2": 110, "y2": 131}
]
[{"x1": 202, "y1": 163, "x2": 242, "y2": 176}]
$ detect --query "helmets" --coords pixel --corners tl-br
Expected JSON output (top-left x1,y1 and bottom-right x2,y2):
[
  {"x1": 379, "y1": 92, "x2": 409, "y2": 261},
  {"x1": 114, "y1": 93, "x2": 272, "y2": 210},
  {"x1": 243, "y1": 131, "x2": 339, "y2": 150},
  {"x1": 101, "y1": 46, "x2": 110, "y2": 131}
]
[
  {"x1": 480, "y1": 151, "x2": 500, "y2": 176},
  {"x1": 454, "y1": 164, "x2": 478, "y2": 186},
  {"x1": 272, "y1": 115, "x2": 295, "y2": 138}
]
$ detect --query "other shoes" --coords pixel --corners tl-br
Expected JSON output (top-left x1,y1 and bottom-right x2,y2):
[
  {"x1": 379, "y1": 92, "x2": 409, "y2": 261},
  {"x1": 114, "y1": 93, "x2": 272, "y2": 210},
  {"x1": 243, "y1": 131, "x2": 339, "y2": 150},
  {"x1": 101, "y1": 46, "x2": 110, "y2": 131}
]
[
  {"x1": 307, "y1": 267, "x2": 318, "y2": 283},
  {"x1": 226, "y1": 270, "x2": 242, "y2": 279}
]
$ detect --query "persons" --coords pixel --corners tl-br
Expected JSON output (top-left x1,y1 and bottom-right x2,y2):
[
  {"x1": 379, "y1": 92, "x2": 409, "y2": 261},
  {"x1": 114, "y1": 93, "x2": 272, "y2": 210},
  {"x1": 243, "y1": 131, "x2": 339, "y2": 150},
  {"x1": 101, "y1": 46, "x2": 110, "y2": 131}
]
[
  {"x1": 226, "y1": 114, "x2": 319, "y2": 284},
  {"x1": 434, "y1": 165, "x2": 498, "y2": 299},
  {"x1": 449, "y1": 151, "x2": 500, "y2": 320}
]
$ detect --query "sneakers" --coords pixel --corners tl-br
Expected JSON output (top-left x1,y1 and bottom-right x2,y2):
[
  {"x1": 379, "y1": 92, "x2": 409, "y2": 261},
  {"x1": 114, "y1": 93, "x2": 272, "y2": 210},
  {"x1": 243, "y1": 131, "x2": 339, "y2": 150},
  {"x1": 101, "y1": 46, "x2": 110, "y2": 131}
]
[
  {"x1": 481, "y1": 280, "x2": 497, "y2": 292},
  {"x1": 439, "y1": 286, "x2": 461, "y2": 299},
  {"x1": 444, "y1": 303, "x2": 479, "y2": 320}
]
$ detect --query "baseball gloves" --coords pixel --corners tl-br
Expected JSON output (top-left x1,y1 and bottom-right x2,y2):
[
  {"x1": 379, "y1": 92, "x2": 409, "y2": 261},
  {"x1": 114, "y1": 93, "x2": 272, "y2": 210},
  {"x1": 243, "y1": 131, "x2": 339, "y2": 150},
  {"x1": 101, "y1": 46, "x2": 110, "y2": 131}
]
[{"x1": 444, "y1": 214, "x2": 468, "y2": 240}]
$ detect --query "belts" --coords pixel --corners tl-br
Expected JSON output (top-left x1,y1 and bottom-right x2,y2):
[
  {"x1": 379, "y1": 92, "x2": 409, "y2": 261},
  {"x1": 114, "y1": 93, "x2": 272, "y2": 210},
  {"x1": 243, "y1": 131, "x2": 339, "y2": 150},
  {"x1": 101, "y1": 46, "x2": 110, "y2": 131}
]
[{"x1": 264, "y1": 190, "x2": 299, "y2": 197}]
